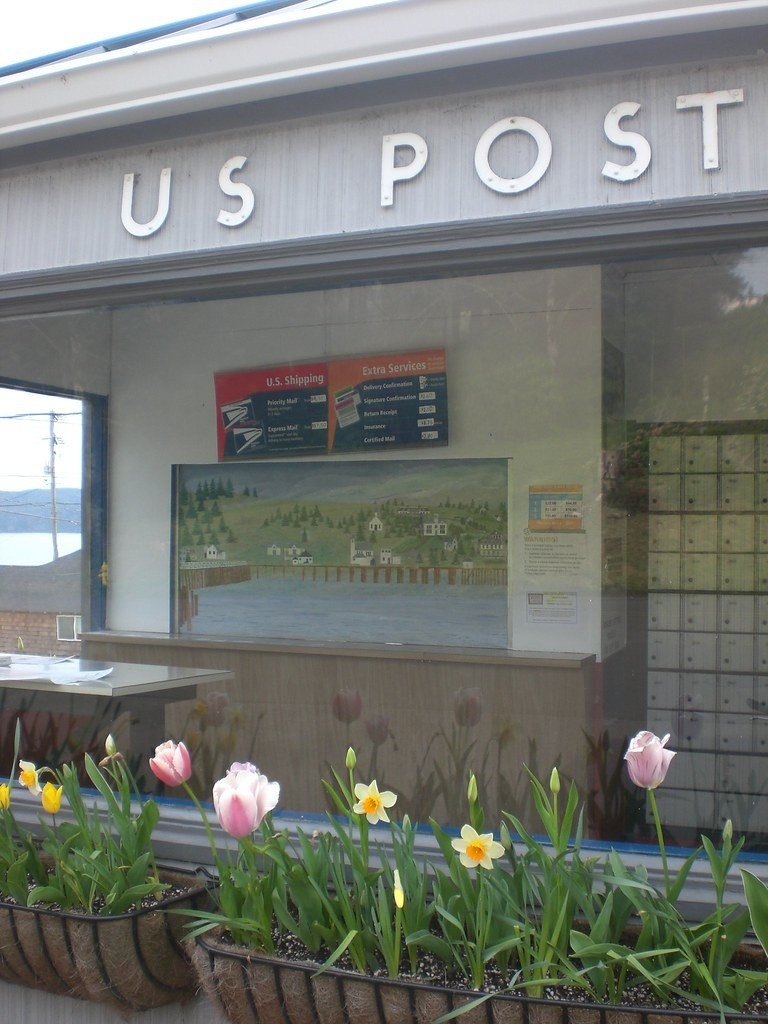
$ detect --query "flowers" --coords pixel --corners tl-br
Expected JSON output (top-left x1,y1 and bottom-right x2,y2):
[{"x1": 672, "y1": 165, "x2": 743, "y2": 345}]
[
  {"x1": 150, "y1": 728, "x2": 768, "y2": 1024},
  {"x1": 322, "y1": 691, "x2": 768, "y2": 854},
  {"x1": 1, "y1": 714, "x2": 172, "y2": 914},
  {"x1": 0, "y1": 684, "x2": 264, "y2": 799}
]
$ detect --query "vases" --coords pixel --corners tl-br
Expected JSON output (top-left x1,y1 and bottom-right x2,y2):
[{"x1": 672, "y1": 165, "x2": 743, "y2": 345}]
[
  {"x1": 188, "y1": 911, "x2": 768, "y2": 1024},
  {"x1": 0, "y1": 850, "x2": 228, "y2": 1010}
]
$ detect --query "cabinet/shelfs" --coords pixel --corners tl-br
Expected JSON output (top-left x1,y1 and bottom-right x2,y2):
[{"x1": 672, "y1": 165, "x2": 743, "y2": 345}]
[{"x1": 626, "y1": 418, "x2": 768, "y2": 853}]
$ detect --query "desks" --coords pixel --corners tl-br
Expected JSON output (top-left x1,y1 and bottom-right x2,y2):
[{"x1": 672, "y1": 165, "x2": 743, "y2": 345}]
[{"x1": 0, "y1": 651, "x2": 236, "y2": 794}]
